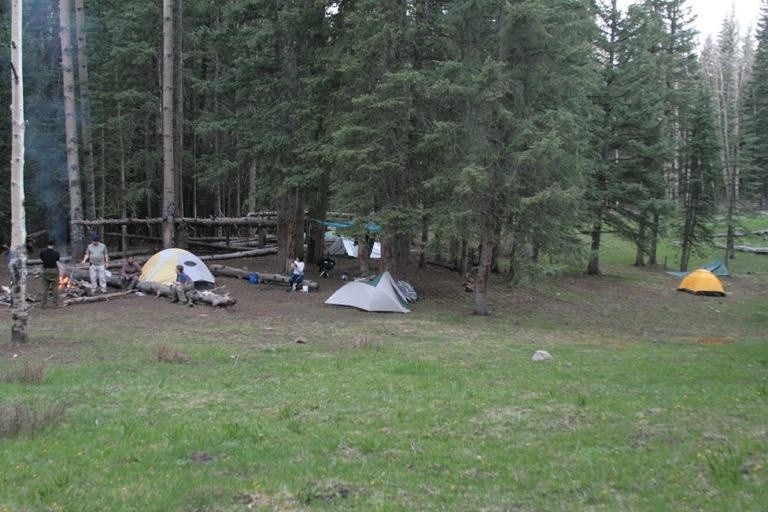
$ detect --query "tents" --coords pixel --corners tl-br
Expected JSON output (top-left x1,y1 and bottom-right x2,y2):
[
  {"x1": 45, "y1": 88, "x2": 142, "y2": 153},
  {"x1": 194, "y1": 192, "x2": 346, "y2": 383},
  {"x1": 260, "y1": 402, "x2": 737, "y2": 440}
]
[
  {"x1": 136, "y1": 246, "x2": 217, "y2": 287},
  {"x1": 678, "y1": 267, "x2": 726, "y2": 298},
  {"x1": 323, "y1": 269, "x2": 413, "y2": 313},
  {"x1": 324, "y1": 234, "x2": 381, "y2": 259}
]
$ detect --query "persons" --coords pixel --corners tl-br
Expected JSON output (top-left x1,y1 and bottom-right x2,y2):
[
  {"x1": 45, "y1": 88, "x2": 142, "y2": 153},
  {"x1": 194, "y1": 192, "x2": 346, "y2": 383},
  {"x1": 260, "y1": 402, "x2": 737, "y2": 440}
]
[
  {"x1": 39, "y1": 239, "x2": 61, "y2": 309},
  {"x1": 81, "y1": 234, "x2": 110, "y2": 293},
  {"x1": 119, "y1": 254, "x2": 143, "y2": 292},
  {"x1": 170, "y1": 264, "x2": 196, "y2": 306},
  {"x1": 286, "y1": 256, "x2": 306, "y2": 292}
]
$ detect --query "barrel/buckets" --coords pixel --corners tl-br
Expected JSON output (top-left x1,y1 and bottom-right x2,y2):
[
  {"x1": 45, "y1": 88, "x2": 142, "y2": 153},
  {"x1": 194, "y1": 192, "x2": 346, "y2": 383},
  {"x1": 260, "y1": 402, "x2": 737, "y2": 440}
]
[{"x1": 249, "y1": 273, "x2": 258, "y2": 284}]
